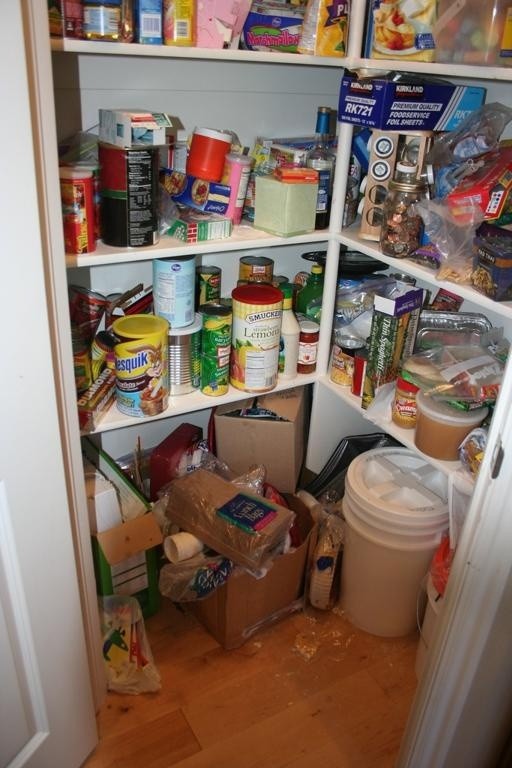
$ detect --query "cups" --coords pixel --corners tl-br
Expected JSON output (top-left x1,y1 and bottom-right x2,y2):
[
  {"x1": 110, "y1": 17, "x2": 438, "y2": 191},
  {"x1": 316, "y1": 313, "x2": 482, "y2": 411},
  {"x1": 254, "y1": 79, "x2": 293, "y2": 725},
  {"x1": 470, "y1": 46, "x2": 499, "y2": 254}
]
[{"x1": 163, "y1": 531, "x2": 205, "y2": 565}]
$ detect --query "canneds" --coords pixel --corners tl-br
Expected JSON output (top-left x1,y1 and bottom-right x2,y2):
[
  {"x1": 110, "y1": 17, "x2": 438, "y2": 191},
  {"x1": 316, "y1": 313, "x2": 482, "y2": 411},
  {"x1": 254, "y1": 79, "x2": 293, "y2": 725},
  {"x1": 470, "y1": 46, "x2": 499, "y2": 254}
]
[{"x1": 69, "y1": 254, "x2": 420, "y2": 430}]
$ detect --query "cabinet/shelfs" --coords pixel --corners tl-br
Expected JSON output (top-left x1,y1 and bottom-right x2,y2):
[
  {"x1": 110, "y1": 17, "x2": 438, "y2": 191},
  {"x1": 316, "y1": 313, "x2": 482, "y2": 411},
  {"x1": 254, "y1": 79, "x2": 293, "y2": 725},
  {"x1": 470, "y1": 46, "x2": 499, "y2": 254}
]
[{"x1": 46, "y1": 0, "x2": 511, "y2": 498}]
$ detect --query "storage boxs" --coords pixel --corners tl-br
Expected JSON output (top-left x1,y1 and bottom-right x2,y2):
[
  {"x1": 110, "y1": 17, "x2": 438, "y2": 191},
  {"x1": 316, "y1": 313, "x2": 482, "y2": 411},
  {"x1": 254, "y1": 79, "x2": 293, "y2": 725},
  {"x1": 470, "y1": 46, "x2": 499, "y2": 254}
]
[
  {"x1": 162, "y1": 496, "x2": 318, "y2": 651},
  {"x1": 165, "y1": 467, "x2": 296, "y2": 571},
  {"x1": 214, "y1": 384, "x2": 311, "y2": 497},
  {"x1": 82, "y1": 436, "x2": 163, "y2": 622}
]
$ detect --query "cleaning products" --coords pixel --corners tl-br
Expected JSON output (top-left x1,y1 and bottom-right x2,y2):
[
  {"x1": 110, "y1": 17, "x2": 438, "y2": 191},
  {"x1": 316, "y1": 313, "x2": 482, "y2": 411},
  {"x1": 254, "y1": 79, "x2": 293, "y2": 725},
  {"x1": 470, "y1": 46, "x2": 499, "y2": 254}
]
[{"x1": 276, "y1": 282, "x2": 304, "y2": 382}]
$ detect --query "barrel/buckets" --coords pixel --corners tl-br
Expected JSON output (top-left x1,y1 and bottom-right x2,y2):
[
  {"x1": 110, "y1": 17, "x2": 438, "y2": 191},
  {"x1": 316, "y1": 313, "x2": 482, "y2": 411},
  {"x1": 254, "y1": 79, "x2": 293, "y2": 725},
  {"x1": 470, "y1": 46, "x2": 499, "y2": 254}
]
[
  {"x1": 415, "y1": 568, "x2": 444, "y2": 681},
  {"x1": 338, "y1": 446, "x2": 449, "y2": 639}
]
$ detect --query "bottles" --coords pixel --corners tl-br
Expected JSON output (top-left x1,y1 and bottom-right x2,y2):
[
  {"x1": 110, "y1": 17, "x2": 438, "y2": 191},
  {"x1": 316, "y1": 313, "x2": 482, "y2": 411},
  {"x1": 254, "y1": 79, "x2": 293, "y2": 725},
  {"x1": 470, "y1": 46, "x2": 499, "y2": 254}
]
[
  {"x1": 380, "y1": 178, "x2": 427, "y2": 259},
  {"x1": 306, "y1": 106, "x2": 335, "y2": 230},
  {"x1": 278, "y1": 282, "x2": 300, "y2": 381}
]
